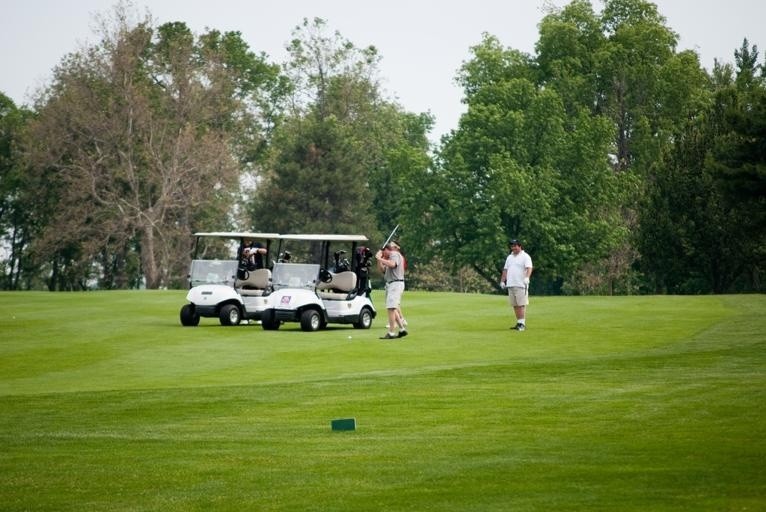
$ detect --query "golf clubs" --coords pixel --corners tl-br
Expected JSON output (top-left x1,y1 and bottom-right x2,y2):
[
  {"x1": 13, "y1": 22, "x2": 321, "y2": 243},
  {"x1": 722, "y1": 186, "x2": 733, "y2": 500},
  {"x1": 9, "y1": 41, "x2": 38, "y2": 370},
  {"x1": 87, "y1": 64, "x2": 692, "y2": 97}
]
[
  {"x1": 381, "y1": 224, "x2": 399, "y2": 250},
  {"x1": 334, "y1": 249, "x2": 349, "y2": 268},
  {"x1": 356, "y1": 246, "x2": 372, "y2": 268}
]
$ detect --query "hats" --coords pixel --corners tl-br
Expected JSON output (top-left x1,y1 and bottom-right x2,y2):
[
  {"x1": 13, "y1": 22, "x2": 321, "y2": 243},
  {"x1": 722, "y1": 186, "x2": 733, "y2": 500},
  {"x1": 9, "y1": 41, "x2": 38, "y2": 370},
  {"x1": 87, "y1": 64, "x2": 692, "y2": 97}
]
[
  {"x1": 379, "y1": 242, "x2": 401, "y2": 250},
  {"x1": 509, "y1": 239, "x2": 519, "y2": 244}
]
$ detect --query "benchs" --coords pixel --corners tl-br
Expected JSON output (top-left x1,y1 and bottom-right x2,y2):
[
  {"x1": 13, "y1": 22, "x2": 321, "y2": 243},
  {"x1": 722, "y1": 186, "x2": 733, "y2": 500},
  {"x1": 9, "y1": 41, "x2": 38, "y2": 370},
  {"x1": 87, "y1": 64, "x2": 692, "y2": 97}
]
[
  {"x1": 235, "y1": 268, "x2": 271, "y2": 297},
  {"x1": 317, "y1": 270, "x2": 358, "y2": 300}
]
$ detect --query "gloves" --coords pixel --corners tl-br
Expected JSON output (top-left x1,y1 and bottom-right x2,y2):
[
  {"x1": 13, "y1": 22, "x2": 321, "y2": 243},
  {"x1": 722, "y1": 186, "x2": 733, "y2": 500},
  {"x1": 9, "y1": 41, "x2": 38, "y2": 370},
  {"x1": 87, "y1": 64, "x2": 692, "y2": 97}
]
[
  {"x1": 524, "y1": 277, "x2": 530, "y2": 284},
  {"x1": 500, "y1": 282, "x2": 505, "y2": 289}
]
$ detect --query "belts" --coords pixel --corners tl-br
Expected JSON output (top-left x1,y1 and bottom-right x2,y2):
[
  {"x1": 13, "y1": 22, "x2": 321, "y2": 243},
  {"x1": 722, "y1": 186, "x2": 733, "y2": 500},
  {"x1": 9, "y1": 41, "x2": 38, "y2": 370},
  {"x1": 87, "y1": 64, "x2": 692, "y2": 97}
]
[{"x1": 388, "y1": 279, "x2": 404, "y2": 284}]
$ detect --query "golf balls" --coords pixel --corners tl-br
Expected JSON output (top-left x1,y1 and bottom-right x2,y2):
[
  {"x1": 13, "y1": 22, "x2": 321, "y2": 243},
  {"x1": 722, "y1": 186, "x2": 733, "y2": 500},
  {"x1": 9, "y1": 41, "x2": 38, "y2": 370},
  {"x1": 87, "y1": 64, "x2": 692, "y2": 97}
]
[{"x1": 348, "y1": 336, "x2": 352, "y2": 339}]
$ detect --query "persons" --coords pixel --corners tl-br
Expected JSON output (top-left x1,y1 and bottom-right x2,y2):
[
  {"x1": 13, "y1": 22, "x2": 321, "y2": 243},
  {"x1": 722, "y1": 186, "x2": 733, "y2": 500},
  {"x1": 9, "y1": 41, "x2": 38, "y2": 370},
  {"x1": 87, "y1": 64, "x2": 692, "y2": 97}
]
[
  {"x1": 375, "y1": 241, "x2": 408, "y2": 339},
  {"x1": 500, "y1": 239, "x2": 533, "y2": 331},
  {"x1": 238, "y1": 239, "x2": 267, "y2": 269}
]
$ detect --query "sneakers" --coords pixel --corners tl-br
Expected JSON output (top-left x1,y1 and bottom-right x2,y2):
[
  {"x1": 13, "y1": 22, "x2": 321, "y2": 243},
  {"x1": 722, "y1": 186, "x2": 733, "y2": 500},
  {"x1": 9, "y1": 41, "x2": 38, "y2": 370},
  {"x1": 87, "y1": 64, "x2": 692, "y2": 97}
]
[
  {"x1": 509, "y1": 323, "x2": 526, "y2": 331},
  {"x1": 386, "y1": 320, "x2": 407, "y2": 328},
  {"x1": 241, "y1": 319, "x2": 262, "y2": 325},
  {"x1": 379, "y1": 330, "x2": 408, "y2": 339}
]
